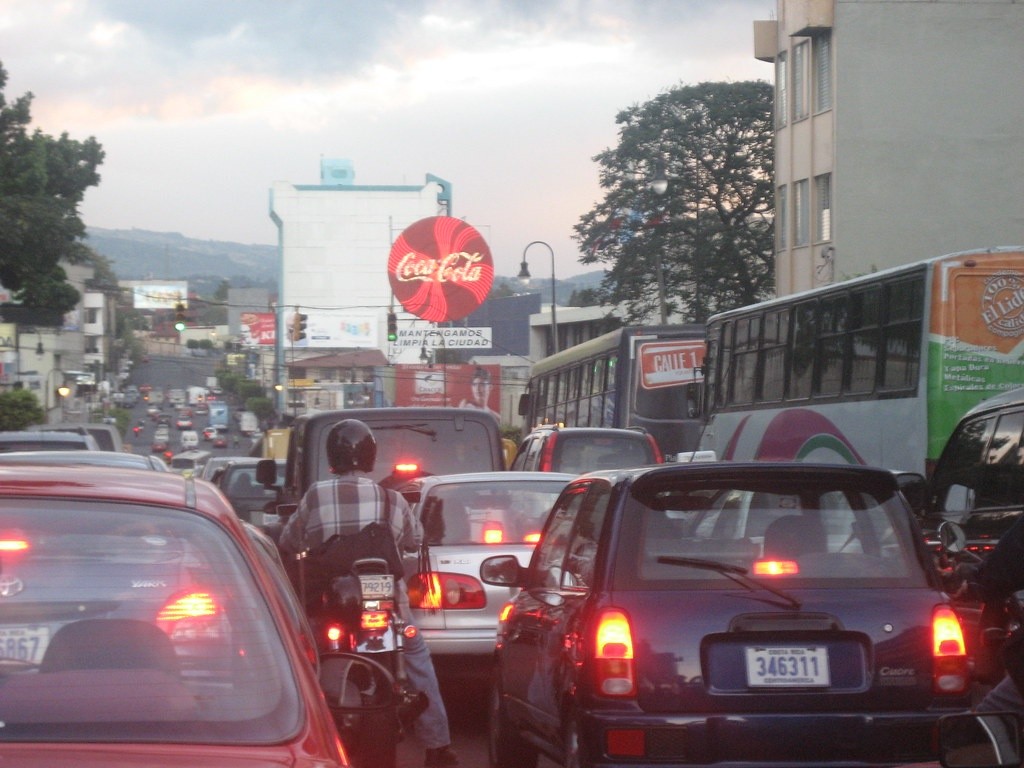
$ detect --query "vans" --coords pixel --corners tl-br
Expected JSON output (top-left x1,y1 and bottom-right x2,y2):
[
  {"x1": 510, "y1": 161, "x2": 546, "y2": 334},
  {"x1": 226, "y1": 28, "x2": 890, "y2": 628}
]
[
  {"x1": 897, "y1": 387, "x2": 1024, "y2": 561},
  {"x1": 254, "y1": 405, "x2": 506, "y2": 539}
]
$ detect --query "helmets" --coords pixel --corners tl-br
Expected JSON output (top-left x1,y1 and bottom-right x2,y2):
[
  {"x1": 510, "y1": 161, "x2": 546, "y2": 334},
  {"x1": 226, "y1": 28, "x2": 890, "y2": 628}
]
[{"x1": 326, "y1": 418, "x2": 377, "y2": 474}]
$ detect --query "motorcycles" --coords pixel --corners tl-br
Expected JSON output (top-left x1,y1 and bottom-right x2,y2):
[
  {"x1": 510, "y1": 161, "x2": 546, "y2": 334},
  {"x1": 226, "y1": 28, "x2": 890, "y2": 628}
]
[{"x1": 306, "y1": 573, "x2": 432, "y2": 768}]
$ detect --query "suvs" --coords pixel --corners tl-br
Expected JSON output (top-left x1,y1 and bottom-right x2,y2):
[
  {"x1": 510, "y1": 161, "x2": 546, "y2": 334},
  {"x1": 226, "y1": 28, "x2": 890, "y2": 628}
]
[{"x1": 507, "y1": 424, "x2": 662, "y2": 481}]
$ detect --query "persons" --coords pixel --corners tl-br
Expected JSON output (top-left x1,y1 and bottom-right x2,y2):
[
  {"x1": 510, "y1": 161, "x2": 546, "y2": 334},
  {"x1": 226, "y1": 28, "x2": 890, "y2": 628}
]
[
  {"x1": 274, "y1": 418, "x2": 456, "y2": 768},
  {"x1": 942, "y1": 513, "x2": 1023, "y2": 714},
  {"x1": 458, "y1": 369, "x2": 500, "y2": 428}
]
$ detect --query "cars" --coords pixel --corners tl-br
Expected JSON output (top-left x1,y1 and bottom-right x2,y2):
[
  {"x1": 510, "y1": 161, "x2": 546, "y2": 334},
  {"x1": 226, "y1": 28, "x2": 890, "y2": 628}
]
[
  {"x1": 119, "y1": 374, "x2": 256, "y2": 455},
  {"x1": 383, "y1": 471, "x2": 593, "y2": 658},
  {"x1": 479, "y1": 461, "x2": 987, "y2": 767},
  {"x1": 1, "y1": 419, "x2": 288, "y2": 536},
  {"x1": 1, "y1": 467, "x2": 352, "y2": 768}
]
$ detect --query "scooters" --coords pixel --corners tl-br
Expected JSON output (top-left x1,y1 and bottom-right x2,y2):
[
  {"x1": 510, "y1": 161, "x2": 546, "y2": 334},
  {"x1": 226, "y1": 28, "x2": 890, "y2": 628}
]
[{"x1": 935, "y1": 521, "x2": 1024, "y2": 707}]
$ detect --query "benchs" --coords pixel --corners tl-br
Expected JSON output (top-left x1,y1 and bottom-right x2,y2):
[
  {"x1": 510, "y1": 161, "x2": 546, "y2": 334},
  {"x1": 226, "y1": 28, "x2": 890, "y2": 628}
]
[{"x1": 635, "y1": 539, "x2": 885, "y2": 580}]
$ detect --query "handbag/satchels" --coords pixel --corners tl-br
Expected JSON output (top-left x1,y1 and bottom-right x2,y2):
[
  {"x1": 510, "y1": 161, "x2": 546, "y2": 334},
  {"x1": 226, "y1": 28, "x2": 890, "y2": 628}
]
[{"x1": 303, "y1": 522, "x2": 407, "y2": 617}]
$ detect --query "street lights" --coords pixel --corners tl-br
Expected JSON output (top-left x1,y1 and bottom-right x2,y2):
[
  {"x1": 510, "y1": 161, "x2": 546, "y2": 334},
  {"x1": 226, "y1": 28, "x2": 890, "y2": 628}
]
[
  {"x1": 516, "y1": 241, "x2": 561, "y2": 353},
  {"x1": 420, "y1": 332, "x2": 450, "y2": 406}
]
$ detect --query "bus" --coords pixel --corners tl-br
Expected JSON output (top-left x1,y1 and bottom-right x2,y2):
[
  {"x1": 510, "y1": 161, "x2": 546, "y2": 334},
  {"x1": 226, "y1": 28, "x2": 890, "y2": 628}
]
[
  {"x1": 517, "y1": 325, "x2": 709, "y2": 461},
  {"x1": 174, "y1": 449, "x2": 217, "y2": 478},
  {"x1": 685, "y1": 247, "x2": 1022, "y2": 480}
]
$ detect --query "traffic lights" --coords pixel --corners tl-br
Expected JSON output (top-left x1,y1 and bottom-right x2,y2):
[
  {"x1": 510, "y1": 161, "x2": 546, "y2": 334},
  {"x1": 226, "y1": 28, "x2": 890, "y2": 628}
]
[
  {"x1": 174, "y1": 304, "x2": 186, "y2": 332},
  {"x1": 292, "y1": 313, "x2": 308, "y2": 342},
  {"x1": 386, "y1": 313, "x2": 398, "y2": 342}
]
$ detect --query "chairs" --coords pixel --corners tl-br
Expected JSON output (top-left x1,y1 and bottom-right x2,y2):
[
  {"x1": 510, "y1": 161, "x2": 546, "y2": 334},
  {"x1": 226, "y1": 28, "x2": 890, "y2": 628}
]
[{"x1": 0, "y1": 617, "x2": 195, "y2": 723}]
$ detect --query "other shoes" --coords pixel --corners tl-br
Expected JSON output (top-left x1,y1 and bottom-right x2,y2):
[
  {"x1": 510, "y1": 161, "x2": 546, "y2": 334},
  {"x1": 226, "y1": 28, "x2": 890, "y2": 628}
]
[{"x1": 425, "y1": 747, "x2": 459, "y2": 767}]
